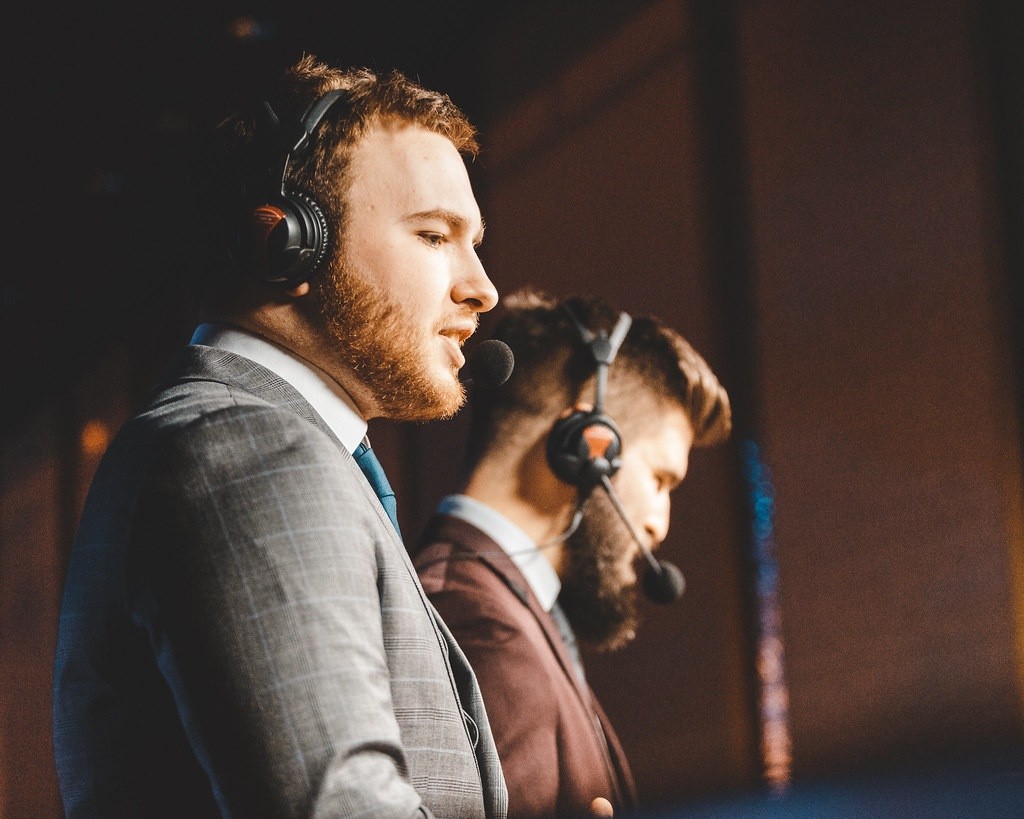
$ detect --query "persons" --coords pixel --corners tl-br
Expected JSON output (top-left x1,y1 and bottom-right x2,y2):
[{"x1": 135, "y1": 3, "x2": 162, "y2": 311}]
[
  {"x1": 414, "y1": 288, "x2": 731, "y2": 819},
  {"x1": 55, "y1": 56, "x2": 513, "y2": 819}
]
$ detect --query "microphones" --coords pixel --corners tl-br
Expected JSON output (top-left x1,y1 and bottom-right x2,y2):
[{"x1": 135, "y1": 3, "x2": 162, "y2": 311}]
[
  {"x1": 467, "y1": 340, "x2": 516, "y2": 390},
  {"x1": 600, "y1": 478, "x2": 686, "y2": 605}
]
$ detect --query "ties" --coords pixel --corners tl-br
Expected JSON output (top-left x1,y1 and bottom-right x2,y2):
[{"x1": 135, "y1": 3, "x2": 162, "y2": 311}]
[
  {"x1": 550, "y1": 601, "x2": 589, "y2": 707},
  {"x1": 352, "y1": 441, "x2": 403, "y2": 546}
]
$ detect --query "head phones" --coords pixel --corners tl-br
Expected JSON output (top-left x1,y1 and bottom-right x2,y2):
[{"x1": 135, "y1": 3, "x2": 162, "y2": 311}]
[
  {"x1": 546, "y1": 303, "x2": 632, "y2": 491},
  {"x1": 227, "y1": 87, "x2": 352, "y2": 285}
]
